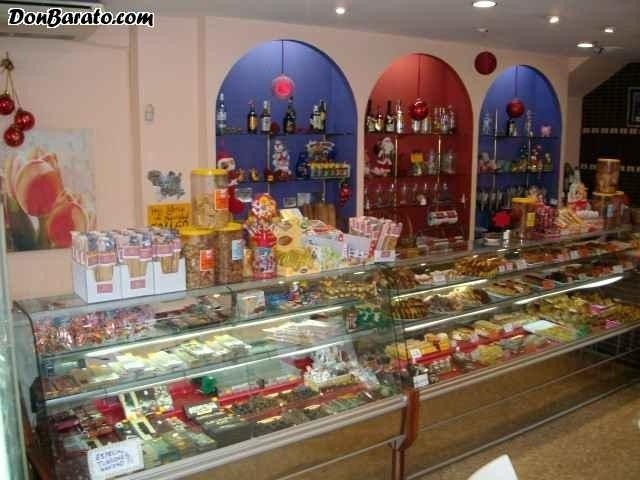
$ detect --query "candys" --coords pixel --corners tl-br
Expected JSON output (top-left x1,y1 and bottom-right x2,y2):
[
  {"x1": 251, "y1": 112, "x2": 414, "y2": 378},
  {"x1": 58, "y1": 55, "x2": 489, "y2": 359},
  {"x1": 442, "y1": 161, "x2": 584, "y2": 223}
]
[{"x1": 243, "y1": 193, "x2": 277, "y2": 237}]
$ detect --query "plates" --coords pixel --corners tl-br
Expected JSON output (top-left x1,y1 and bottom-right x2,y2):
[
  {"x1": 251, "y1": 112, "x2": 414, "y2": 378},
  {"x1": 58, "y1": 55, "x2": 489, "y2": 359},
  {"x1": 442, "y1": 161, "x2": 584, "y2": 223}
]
[{"x1": 392, "y1": 300, "x2": 491, "y2": 323}]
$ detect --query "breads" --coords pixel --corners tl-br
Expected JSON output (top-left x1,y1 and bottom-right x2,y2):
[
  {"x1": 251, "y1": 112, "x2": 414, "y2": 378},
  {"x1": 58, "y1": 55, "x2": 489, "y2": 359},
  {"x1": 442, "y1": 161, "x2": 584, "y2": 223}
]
[
  {"x1": 450, "y1": 254, "x2": 509, "y2": 279},
  {"x1": 362, "y1": 266, "x2": 416, "y2": 290}
]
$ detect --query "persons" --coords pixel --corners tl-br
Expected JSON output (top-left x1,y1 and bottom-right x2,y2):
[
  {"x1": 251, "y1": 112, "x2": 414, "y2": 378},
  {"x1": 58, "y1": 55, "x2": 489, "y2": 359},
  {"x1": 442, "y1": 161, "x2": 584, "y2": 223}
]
[{"x1": 215, "y1": 138, "x2": 293, "y2": 274}]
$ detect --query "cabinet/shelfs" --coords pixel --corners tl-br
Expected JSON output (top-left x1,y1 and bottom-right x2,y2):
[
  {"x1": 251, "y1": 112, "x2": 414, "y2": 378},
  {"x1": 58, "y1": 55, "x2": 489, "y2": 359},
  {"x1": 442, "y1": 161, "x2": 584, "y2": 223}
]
[
  {"x1": 366, "y1": 128, "x2": 457, "y2": 240},
  {"x1": 8, "y1": 260, "x2": 409, "y2": 479},
  {"x1": 214, "y1": 128, "x2": 351, "y2": 234},
  {"x1": 379, "y1": 226, "x2": 639, "y2": 480},
  {"x1": 477, "y1": 135, "x2": 554, "y2": 232}
]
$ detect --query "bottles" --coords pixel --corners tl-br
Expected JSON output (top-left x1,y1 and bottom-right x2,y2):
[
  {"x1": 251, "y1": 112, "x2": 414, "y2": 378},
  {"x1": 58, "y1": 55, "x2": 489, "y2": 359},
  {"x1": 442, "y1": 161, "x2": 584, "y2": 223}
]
[
  {"x1": 510, "y1": 198, "x2": 537, "y2": 243},
  {"x1": 365, "y1": 97, "x2": 457, "y2": 134},
  {"x1": 480, "y1": 112, "x2": 493, "y2": 135},
  {"x1": 363, "y1": 182, "x2": 453, "y2": 207},
  {"x1": 215, "y1": 90, "x2": 329, "y2": 133}
]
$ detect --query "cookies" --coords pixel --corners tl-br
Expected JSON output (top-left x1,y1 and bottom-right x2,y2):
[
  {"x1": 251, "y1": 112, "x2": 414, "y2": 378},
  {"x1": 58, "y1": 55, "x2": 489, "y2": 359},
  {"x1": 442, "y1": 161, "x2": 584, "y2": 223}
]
[{"x1": 388, "y1": 299, "x2": 429, "y2": 320}]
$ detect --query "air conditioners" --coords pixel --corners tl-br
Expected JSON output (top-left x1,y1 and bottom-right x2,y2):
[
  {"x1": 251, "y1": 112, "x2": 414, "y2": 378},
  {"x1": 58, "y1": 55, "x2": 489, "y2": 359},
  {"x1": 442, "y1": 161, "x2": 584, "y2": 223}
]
[{"x1": 1, "y1": 0, "x2": 108, "y2": 41}]
